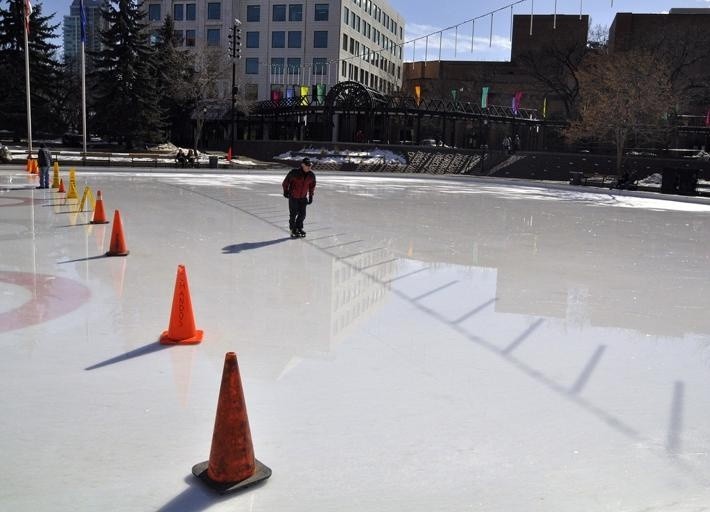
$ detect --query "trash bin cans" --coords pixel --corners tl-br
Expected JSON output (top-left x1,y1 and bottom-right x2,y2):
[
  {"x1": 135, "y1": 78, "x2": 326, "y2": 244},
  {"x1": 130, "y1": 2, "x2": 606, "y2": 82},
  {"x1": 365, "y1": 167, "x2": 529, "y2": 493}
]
[
  {"x1": 568, "y1": 172, "x2": 583, "y2": 186},
  {"x1": 209, "y1": 156, "x2": 218, "y2": 168}
]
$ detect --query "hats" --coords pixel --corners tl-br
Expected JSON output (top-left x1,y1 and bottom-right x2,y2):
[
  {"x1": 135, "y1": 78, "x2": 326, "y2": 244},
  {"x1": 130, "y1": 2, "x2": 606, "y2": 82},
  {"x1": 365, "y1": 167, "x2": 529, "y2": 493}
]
[{"x1": 302, "y1": 158, "x2": 312, "y2": 164}]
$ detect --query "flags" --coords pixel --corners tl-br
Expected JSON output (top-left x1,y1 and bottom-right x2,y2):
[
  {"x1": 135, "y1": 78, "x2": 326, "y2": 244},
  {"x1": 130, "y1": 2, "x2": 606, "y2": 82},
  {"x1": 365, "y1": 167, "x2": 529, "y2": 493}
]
[
  {"x1": 79, "y1": 0, "x2": 87, "y2": 47},
  {"x1": 24, "y1": 0, "x2": 34, "y2": 38}
]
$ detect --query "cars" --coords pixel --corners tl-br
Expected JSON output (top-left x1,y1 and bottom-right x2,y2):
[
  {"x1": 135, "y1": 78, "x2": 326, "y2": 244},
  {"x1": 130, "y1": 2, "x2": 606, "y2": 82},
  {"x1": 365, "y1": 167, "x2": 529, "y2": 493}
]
[{"x1": 419, "y1": 138, "x2": 451, "y2": 148}]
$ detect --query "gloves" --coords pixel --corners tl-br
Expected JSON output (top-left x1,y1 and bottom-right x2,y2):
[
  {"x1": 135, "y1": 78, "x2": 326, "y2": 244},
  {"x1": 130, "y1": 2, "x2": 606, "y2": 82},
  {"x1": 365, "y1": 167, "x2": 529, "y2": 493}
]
[
  {"x1": 284, "y1": 191, "x2": 290, "y2": 198},
  {"x1": 307, "y1": 197, "x2": 312, "y2": 204}
]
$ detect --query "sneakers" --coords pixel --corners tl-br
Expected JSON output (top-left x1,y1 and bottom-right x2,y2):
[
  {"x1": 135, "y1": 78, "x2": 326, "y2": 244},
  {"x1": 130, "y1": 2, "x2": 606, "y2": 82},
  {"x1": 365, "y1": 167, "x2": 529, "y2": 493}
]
[{"x1": 290, "y1": 229, "x2": 306, "y2": 235}]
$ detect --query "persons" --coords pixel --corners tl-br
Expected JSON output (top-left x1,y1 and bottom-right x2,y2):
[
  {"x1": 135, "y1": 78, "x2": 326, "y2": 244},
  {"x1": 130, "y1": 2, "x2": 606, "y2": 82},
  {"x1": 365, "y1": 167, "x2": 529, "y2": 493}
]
[
  {"x1": 175, "y1": 149, "x2": 187, "y2": 168},
  {"x1": 36, "y1": 143, "x2": 54, "y2": 189},
  {"x1": 187, "y1": 149, "x2": 195, "y2": 169},
  {"x1": 281, "y1": 157, "x2": 317, "y2": 238}
]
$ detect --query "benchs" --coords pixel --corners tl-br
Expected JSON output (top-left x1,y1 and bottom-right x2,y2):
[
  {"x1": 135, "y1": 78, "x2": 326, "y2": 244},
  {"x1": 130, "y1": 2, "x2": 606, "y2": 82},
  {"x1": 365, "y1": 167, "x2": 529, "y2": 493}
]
[
  {"x1": 175, "y1": 155, "x2": 200, "y2": 168},
  {"x1": 80, "y1": 152, "x2": 112, "y2": 166},
  {"x1": 25, "y1": 152, "x2": 60, "y2": 165},
  {"x1": 129, "y1": 155, "x2": 159, "y2": 167}
]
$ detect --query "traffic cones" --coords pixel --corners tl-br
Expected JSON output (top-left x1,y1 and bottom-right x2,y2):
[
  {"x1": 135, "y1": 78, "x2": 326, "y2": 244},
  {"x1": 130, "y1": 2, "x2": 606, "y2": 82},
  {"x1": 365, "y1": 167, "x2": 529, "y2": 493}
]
[
  {"x1": 105, "y1": 208, "x2": 131, "y2": 257},
  {"x1": 57, "y1": 179, "x2": 66, "y2": 193},
  {"x1": 66, "y1": 166, "x2": 81, "y2": 199},
  {"x1": 51, "y1": 161, "x2": 62, "y2": 187},
  {"x1": 27, "y1": 159, "x2": 40, "y2": 175},
  {"x1": 159, "y1": 264, "x2": 205, "y2": 346},
  {"x1": 90, "y1": 189, "x2": 109, "y2": 224},
  {"x1": 190, "y1": 351, "x2": 274, "y2": 496},
  {"x1": 227, "y1": 145, "x2": 233, "y2": 160}
]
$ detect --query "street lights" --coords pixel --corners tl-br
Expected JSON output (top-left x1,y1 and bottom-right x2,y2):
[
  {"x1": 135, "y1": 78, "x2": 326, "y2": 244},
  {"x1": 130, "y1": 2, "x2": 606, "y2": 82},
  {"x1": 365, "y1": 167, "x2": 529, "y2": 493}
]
[{"x1": 226, "y1": 16, "x2": 242, "y2": 155}]
